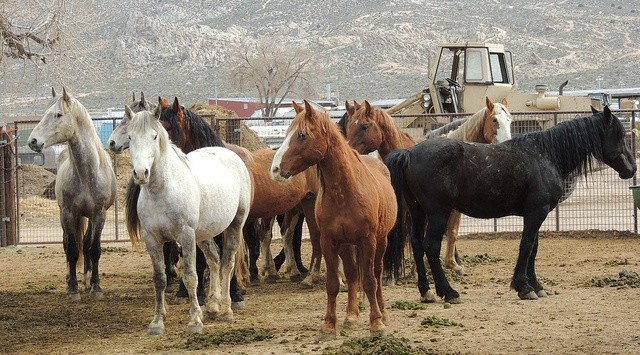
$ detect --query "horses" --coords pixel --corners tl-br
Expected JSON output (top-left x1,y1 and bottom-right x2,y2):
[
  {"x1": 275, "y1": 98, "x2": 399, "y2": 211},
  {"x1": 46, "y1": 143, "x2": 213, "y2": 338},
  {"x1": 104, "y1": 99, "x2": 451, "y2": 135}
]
[
  {"x1": 418, "y1": 95, "x2": 513, "y2": 277},
  {"x1": 107, "y1": 90, "x2": 181, "y2": 282},
  {"x1": 343, "y1": 100, "x2": 418, "y2": 286},
  {"x1": 26, "y1": 86, "x2": 117, "y2": 305},
  {"x1": 153, "y1": 95, "x2": 323, "y2": 288},
  {"x1": 382, "y1": 103, "x2": 638, "y2": 304},
  {"x1": 124, "y1": 104, "x2": 252, "y2": 335},
  {"x1": 269, "y1": 99, "x2": 399, "y2": 340}
]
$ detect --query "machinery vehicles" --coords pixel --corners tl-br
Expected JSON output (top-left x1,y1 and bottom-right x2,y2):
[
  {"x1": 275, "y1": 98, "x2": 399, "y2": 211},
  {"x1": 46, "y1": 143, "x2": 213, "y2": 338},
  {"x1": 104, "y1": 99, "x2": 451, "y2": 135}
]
[{"x1": 387, "y1": 42, "x2": 608, "y2": 148}]
